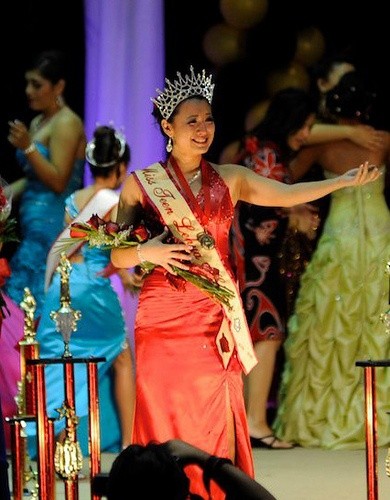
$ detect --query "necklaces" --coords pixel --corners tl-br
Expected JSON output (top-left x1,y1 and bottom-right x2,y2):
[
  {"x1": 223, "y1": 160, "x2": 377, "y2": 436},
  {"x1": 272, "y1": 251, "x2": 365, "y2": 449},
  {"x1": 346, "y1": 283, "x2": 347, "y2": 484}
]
[{"x1": 188, "y1": 168, "x2": 201, "y2": 184}]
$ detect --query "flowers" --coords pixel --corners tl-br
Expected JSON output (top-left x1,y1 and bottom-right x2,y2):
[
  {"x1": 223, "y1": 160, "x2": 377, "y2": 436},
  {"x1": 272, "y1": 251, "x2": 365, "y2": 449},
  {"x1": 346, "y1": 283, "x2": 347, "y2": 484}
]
[
  {"x1": 55, "y1": 215, "x2": 234, "y2": 308},
  {"x1": 0, "y1": 177, "x2": 21, "y2": 251}
]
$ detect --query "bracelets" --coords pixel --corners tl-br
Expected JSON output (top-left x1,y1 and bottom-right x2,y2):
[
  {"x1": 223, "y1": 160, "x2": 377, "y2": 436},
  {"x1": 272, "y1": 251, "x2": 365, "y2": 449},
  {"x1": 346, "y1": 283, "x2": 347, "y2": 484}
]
[
  {"x1": 24, "y1": 145, "x2": 35, "y2": 155},
  {"x1": 137, "y1": 244, "x2": 143, "y2": 262},
  {"x1": 295, "y1": 217, "x2": 299, "y2": 226}
]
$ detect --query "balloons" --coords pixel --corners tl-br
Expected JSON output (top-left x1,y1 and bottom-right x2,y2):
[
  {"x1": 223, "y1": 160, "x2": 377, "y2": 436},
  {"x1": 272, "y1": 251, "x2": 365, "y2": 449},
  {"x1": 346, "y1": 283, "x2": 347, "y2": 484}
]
[
  {"x1": 203, "y1": 0, "x2": 268, "y2": 65},
  {"x1": 245, "y1": 101, "x2": 270, "y2": 132},
  {"x1": 268, "y1": 27, "x2": 325, "y2": 95}
]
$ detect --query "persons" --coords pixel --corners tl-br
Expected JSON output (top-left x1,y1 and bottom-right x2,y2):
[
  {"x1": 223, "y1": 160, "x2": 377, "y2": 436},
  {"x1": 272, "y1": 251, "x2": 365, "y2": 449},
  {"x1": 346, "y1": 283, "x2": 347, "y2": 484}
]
[
  {"x1": 27, "y1": 127, "x2": 135, "y2": 460},
  {"x1": 110, "y1": 65, "x2": 383, "y2": 482},
  {"x1": 0, "y1": 57, "x2": 89, "y2": 328},
  {"x1": 221, "y1": 61, "x2": 390, "y2": 450}
]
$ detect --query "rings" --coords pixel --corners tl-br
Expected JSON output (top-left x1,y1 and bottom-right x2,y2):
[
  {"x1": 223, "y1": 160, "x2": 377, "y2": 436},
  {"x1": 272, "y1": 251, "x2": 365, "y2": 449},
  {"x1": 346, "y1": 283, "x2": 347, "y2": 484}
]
[{"x1": 313, "y1": 227, "x2": 317, "y2": 230}]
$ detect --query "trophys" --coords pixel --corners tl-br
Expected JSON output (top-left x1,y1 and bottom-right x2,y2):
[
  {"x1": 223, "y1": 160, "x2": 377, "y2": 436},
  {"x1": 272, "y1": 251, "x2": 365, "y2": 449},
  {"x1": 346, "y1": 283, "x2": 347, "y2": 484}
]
[
  {"x1": 25, "y1": 251, "x2": 107, "y2": 500},
  {"x1": 0, "y1": 287, "x2": 39, "y2": 500}
]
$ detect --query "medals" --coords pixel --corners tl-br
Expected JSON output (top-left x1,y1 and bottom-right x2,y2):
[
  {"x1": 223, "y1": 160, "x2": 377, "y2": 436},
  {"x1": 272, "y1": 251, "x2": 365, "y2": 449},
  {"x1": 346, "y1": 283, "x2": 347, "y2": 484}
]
[{"x1": 200, "y1": 235, "x2": 215, "y2": 250}]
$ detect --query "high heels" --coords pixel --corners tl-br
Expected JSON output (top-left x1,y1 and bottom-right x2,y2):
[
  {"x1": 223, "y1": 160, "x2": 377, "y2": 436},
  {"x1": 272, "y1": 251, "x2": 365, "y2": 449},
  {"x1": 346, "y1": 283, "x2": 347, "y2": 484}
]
[{"x1": 249, "y1": 435, "x2": 295, "y2": 449}]
